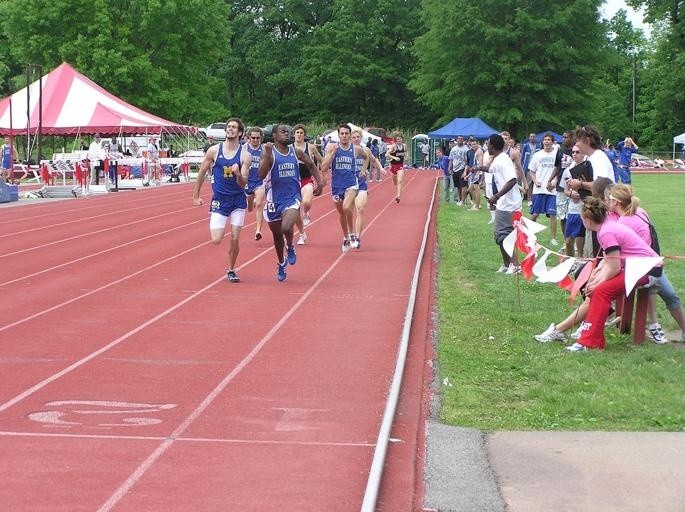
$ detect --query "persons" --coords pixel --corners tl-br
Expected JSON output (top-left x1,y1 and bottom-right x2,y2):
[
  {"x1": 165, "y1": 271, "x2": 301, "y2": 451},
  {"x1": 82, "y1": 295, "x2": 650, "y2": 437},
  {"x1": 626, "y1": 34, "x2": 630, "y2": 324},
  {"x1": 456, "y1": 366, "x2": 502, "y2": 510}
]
[
  {"x1": 201, "y1": 134, "x2": 217, "y2": 180},
  {"x1": 235, "y1": 123, "x2": 388, "y2": 253},
  {"x1": 418, "y1": 128, "x2": 684, "y2": 353},
  {"x1": 191, "y1": 116, "x2": 252, "y2": 283},
  {"x1": 384, "y1": 132, "x2": 409, "y2": 205},
  {"x1": 0, "y1": 136, "x2": 19, "y2": 169},
  {"x1": 258, "y1": 124, "x2": 328, "y2": 282},
  {"x1": 87, "y1": 133, "x2": 162, "y2": 188}
]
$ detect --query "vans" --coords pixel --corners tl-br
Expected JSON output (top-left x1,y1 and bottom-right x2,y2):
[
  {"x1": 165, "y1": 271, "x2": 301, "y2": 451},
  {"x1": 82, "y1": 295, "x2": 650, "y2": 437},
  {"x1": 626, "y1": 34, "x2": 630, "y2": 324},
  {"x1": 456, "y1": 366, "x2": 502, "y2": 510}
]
[{"x1": 99, "y1": 137, "x2": 175, "y2": 159}]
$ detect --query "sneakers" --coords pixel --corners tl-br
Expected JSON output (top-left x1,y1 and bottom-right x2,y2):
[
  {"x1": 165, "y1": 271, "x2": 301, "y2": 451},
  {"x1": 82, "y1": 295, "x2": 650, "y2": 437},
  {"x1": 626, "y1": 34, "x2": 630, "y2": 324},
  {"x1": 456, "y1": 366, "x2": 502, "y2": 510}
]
[
  {"x1": 567, "y1": 320, "x2": 593, "y2": 340},
  {"x1": 395, "y1": 196, "x2": 401, "y2": 204},
  {"x1": 505, "y1": 262, "x2": 523, "y2": 277},
  {"x1": 564, "y1": 342, "x2": 594, "y2": 352},
  {"x1": 454, "y1": 200, "x2": 560, "y2": 247},
  {"x1": 497, "y1": 263, "x2": 509, "y2": 274},
  {"x1": 225, "y1": 268, "x2": 240, "y2": 283},
  {"x1": 284, "y1": 241, "x2": 298, "y2": 266},
  {"x1": 254, "y1": 230, "x2": 264, "y2": 242},
  {"x1": 648, "y1": 321, "x2": 669, "y2": 345},
  {"x1": 296, "y1": 230, "x2": 308, "y2": 246},
  {"x1": 302, "y1": 212, "x2": 311, "y2": 228},
  {"x1": 277, "y1": 255, "x2": 289, "y2": 283},
  {"x1": 533, "y1": 321, "x2": 567, "y2": 345},
  {"x1": 341, "y1": 233, "x2": 362, "y2": 253}
]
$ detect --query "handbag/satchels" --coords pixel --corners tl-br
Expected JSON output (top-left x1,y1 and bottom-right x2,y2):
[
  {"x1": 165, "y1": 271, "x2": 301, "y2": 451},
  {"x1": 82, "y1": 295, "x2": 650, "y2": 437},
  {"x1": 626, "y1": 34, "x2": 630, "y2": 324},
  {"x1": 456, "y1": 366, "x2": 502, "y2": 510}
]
[{"x1": 568, "y1": 160, "x2": 594, "y2": 202}]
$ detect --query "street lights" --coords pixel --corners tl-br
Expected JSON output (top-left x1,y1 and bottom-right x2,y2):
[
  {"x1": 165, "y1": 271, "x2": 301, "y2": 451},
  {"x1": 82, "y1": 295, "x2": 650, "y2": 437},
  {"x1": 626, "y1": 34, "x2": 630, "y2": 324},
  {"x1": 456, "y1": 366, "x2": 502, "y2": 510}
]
[{"x1": 628, "y1": 47, "x2": 640, "y2": 143}]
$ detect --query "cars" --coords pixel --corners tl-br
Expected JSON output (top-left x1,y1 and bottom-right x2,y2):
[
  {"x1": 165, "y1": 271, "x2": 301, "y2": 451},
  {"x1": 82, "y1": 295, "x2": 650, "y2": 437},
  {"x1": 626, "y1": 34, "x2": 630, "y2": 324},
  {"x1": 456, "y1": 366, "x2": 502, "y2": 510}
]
[
  {"x1": 364, "y1": 124, "x2": 395, "y2": 145},
  {"x1": 192, "y1": 121, "x2": 246, "y2": 142},
  {"x1": 261, "y1": 124, "x2": 310, "y2": 145},
  {"x1": 632, "y1": 153, "x2": 653, "y2": 166}
]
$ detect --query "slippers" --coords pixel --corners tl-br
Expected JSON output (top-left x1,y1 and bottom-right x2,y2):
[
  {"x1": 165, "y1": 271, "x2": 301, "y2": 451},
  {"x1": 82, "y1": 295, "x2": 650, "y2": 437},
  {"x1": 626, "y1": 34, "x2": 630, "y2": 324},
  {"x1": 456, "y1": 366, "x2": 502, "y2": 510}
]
[{"x1": 603, "y1": 316, "x2": 622, "y2": 329}]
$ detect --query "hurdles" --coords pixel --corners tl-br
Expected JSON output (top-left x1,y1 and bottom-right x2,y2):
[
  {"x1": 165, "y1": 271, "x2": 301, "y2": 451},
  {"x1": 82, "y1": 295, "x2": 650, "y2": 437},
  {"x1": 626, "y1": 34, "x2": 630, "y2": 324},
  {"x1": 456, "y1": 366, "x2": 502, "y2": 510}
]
[{"x1": 38, "y1": 151, "x2": 211, "y2": 198}]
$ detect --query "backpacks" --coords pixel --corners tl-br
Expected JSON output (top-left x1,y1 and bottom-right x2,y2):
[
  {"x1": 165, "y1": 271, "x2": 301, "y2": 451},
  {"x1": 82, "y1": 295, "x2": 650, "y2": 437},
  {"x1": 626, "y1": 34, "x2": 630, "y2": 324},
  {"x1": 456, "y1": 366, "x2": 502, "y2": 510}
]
[{"x1": 632, "y1": 211, "x2": 664, "y2": 278}]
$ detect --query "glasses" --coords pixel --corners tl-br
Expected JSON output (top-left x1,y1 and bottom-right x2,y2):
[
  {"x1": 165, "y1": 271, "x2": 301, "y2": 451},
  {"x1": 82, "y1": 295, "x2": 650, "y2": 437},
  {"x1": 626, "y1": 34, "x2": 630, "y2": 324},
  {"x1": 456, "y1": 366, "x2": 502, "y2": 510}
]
[
  {"x1": 250, "y1": 137, "x2": 260, "y2": 139},
  {"x1": 607, "y1": 193, "x2": 621, "y2": 203}
]
